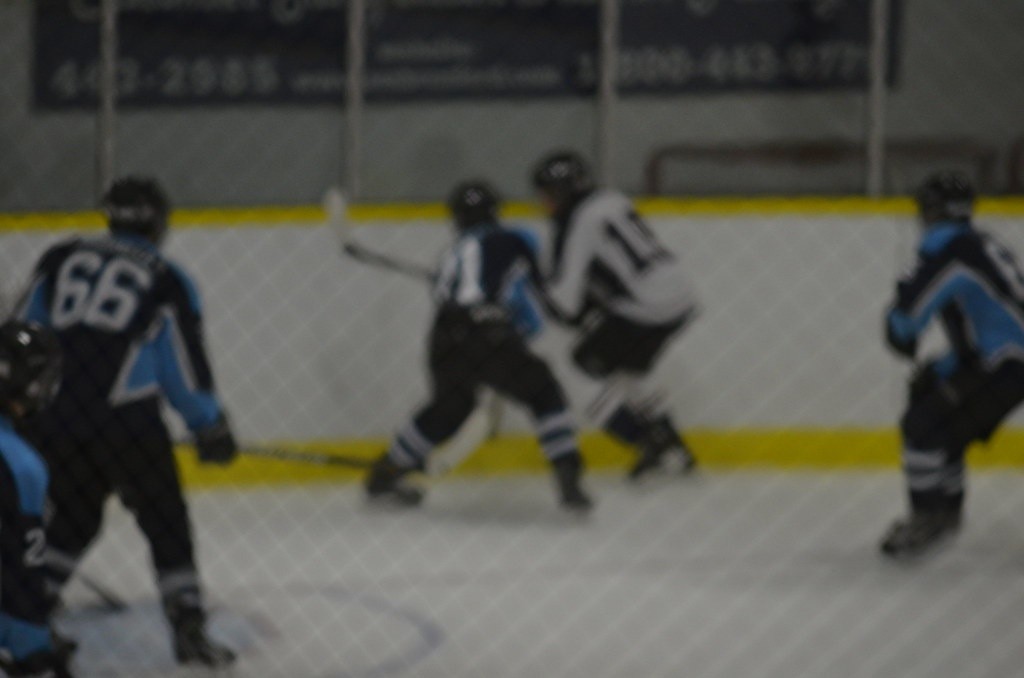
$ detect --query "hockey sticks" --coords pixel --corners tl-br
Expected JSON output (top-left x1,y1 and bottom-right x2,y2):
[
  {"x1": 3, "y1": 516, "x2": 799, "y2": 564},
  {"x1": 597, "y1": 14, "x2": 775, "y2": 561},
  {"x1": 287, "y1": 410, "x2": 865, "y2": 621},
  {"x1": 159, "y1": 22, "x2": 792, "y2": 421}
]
[
  {"x1": 324, "y1": 185, "x2": 430, "y2": 276},
  {"x1": 169, "y1": 431, "x2": 375, "y2": 472},
  {"x1": 75, "y1": 562, "x2": 135, "y2": 611}
]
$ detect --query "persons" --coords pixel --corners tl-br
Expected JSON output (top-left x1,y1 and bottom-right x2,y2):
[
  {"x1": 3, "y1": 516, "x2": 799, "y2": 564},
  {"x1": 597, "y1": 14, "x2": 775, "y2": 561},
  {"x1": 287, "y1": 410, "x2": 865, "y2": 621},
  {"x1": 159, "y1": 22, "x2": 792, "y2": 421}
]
[
  {"x1": 533, "y1": 153, "x2": 703, "y2": 492},
  {"x1": 0, "y1": 173, "x2": 244, "y2": 678},
  {"x1": 363, "y1": 177, "x2": 592, "y2": 512},
  {"x1": 878, "y1": 165, "x2": 1024, "y2": 564}
]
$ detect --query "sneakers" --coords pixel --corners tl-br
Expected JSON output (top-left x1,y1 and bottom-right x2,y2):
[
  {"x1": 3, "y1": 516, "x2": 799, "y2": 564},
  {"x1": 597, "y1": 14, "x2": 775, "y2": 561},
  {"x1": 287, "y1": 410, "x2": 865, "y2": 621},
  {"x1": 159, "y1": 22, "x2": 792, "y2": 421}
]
[
  {"x1": 881, "y1": 489, "x2": 963, "y2": 559},
  {"x1": 169, "y1": 604, "x2": 237, "y2": 668},
  {"x1": 625, "y1": 412, "x2": 697, "y2": 487},
  {"x1": 546, "y1": 449, "x2": 598, "y2": 515},
  {"x1": 360, "y1": 451, "x2": 425, "y2": 509}
]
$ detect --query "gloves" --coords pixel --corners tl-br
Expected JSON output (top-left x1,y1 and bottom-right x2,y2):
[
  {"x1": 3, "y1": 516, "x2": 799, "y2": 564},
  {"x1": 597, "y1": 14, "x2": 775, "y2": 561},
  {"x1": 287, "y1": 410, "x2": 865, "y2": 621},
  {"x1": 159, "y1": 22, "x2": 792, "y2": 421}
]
[
  {"x1": 195, "y1": 428, "x2": 240, "y2": 463},
  {"x1": 887, "y1": 327, "x2": 918, "y2": 357}
]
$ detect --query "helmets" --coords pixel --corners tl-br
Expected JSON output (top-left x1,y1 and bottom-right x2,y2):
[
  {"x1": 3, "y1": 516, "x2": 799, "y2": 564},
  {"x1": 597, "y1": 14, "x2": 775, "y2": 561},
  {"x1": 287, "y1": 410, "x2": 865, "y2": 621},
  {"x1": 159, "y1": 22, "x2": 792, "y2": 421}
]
[
  {"x1": 448, "y1": 178, "x2": 497, "y2": 221},
  {"x1": 102, "y1": 179, "x2": 168, "y2": 237},
  {"x1": 914, "y1": 171, "x2": 980, "y2": 220},
  {"x1": 528, "y1": 151, "x2": 592, "y2": 198}
]
